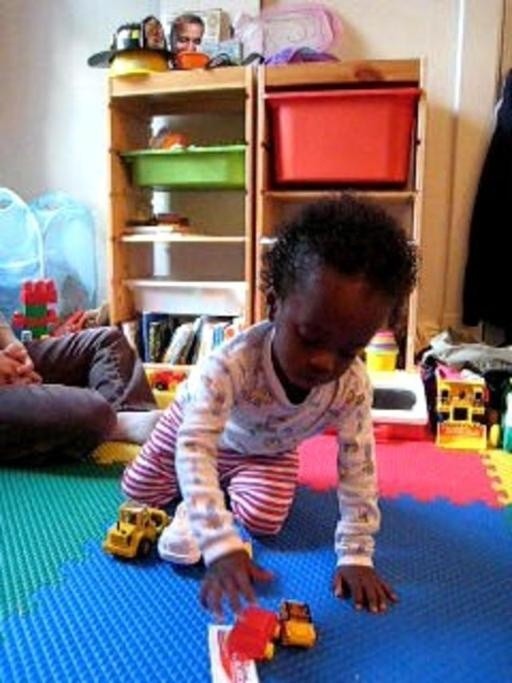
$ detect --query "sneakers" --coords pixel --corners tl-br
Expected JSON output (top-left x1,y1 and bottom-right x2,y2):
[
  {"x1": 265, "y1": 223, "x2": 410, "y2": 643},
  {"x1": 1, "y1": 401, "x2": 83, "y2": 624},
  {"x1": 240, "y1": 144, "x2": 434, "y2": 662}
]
[{"x1": 157, "y1": 499, "x2": 202, "y2": 566}]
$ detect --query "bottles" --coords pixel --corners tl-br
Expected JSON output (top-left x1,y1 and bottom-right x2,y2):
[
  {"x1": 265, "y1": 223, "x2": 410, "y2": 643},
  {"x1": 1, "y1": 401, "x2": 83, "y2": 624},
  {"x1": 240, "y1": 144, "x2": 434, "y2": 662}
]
[
  {"x1": 502, "y1": 378, "x2": 511, "y2": 453},
  {"x1": 361, "y1": 330, "x2": 399, "y2": 373}
]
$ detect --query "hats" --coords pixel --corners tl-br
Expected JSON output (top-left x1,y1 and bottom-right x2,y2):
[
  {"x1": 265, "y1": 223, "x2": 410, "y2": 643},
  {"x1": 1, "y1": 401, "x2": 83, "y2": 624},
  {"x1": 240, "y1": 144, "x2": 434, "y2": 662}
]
[{"x1": 88, "y1": 24, "x2": 175, "y2": 74}]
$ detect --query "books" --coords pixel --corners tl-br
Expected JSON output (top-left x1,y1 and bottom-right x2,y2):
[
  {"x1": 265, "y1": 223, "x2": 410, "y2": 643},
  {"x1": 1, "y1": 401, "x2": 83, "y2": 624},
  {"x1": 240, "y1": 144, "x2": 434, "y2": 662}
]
[{"x1": 123, "y1": 312, "x2": 242, "y2": 363}]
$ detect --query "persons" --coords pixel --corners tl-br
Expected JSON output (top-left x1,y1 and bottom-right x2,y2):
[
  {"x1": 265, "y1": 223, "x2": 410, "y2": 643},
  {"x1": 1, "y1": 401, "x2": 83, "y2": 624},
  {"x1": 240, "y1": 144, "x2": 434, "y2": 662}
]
[
  {"x1": 0, "y1": 310, "x2": 167, "y2": 467},
  {"x1": 121, "y1": 193, "x2": 418, "y2": 620},
  {"x1": 170, "y1": 13, "x2": 206, "y2": 65}
]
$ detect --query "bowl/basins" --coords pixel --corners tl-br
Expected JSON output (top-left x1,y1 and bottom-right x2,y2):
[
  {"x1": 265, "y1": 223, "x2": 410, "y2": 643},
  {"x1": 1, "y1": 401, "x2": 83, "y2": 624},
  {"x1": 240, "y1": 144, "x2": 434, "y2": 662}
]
[
  {"x1": 175, "y1": 52, "x2": 209, "y2": 70},
  {"x1": 110, "y1": 51, "x2": 169, "y2": 73}
]
